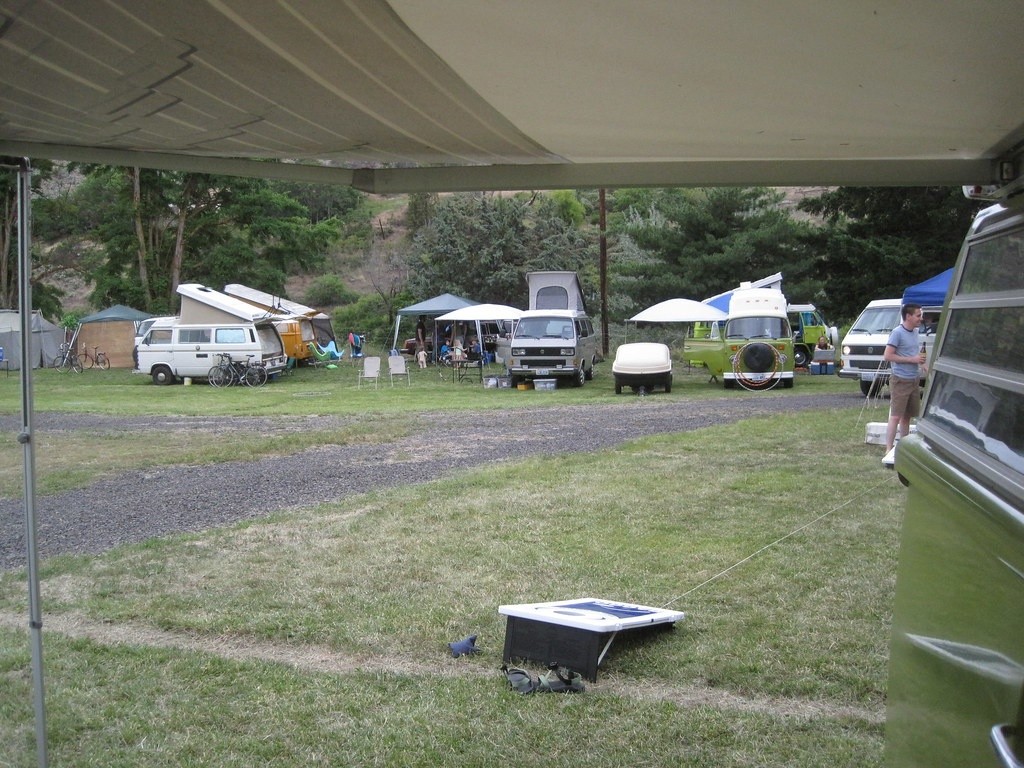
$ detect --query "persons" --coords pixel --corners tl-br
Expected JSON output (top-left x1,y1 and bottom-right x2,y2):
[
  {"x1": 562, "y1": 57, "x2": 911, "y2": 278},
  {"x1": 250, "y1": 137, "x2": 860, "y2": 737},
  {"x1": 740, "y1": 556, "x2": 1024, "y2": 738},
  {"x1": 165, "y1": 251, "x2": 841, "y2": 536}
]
[
  {"x1": 414, "y1": 315, "x2": 428, "y2": 368},
  {"x1": 814, "y1": 337, "x2": 835, "y2": 363},
  {"x1": 884, "y1": 304, "x2": 924, "y2": 469},
  {"x1": 439, "y1": 338, "x2": 480, "y2": 365}
]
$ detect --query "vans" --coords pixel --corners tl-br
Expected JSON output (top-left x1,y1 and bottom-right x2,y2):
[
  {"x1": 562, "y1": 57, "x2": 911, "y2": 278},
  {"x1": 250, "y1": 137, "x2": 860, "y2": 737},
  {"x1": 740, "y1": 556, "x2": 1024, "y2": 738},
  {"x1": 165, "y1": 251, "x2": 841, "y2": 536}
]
[
  {"x1": 507, "y1": 309, "x2": 596, "y2": 388},
  {"x1": 686, "y1": 303, "x2": 830, "y2": 368},
  {"x1": 683, "y1": 280, "x2": 795, "y2": 389},
  {"x1": 837, "y1": 298, "x2": 946, "y2": 399},
  {"x1": 130, "y1": 292, "x2": 293, "y2": 385}
]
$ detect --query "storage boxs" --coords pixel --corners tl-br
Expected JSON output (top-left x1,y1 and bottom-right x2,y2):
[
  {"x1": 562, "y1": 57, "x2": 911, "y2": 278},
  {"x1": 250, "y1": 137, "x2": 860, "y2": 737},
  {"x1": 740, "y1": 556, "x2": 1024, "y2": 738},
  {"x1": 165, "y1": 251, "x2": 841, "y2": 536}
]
[
  {"x1": 517, "y1": 380, "x2": 534, "y2": 391},
  {"x1": 810, "y1": 361, "x2": 835, "y2": 375},
  {"x1": 497, "y1": 374, "x2": 516, "y2": 388},
  {"x1": 483, "y1": 373, "x2": 501, "y2": 389},
  {"x1": 864, "y1": 421, "x2": 917, "y2": 446},
  {"x1": 532, "y1": 378, "x2": 557, "y2": 392}
]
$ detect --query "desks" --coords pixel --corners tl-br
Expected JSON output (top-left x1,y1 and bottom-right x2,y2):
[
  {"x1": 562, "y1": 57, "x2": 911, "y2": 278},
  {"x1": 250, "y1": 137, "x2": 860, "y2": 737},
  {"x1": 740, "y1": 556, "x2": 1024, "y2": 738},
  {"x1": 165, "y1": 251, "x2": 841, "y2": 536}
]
[{"x1": 450, "y1": 359, "x2": 469, "y2": 383}]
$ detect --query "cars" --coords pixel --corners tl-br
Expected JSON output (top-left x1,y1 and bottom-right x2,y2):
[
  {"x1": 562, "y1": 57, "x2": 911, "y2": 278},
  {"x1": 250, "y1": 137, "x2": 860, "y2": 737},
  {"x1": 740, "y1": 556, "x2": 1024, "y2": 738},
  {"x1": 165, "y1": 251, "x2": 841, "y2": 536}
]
[{"x1": 401, "y1": 320, "x2": 526, "y2": 361}]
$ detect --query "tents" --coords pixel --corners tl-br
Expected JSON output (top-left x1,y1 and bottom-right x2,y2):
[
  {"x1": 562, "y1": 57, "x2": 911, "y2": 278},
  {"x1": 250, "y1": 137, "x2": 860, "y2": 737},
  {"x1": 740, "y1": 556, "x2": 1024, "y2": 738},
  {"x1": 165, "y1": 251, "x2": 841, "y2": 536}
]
[
  {"x1": 392, "y1": 293, "x2": 483, "y2": 350},
  {"x1": 77, "y1": 304, "x2": 152, "y2": 368},
  {"x1": 624, "y1": 298, "x2": 729, "y2": 344},
  {"x1": 903, "y1": 267, "x2": 954, "y2": 307},
  {"x1": 434, "y1": 304, "x2": 524, "y2": 367},
  {"x1": 0, "y1": 308, "x2": 65, "y2": 370}
]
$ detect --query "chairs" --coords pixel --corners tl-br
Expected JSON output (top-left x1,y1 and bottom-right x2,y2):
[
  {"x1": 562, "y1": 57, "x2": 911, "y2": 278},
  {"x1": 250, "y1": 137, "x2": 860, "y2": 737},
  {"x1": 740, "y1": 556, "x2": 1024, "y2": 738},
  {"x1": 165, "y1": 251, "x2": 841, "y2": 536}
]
[
  {"x1": 357, "y1": 356, "x2": 381, "y2": 391},
  {"x1": 388, "y1": 355, "x2": 410, "y2": 388},
  {"x1": 308, "y1": 341, "x2": 331, "y2": 370},
  {"x1": 348, "y1": 333, "x2": 366, "y2": 368},
  {"x1": 281, "y1": 357, "x2": 296, "y2": 376},
  {"x1": 316, "y1": 340, "x2": 345, "y2": 362},
  {"x1": 562, "y1": 326, "x2": 573, "y2": 337}
]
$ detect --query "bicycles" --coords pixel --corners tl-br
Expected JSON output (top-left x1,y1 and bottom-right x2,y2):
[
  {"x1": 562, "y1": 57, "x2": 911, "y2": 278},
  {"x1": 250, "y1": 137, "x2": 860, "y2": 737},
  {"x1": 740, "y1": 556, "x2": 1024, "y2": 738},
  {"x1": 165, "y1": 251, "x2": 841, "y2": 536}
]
[
  {"x1": 53, "y1": 343, "x2": 83, "y2": 376},
  {"x1": 76, "y1": 342, "x2": 110, "y2": 371},
  {"x1": 208, "y1": 353, "x2": 268, "y2": 388}
]
